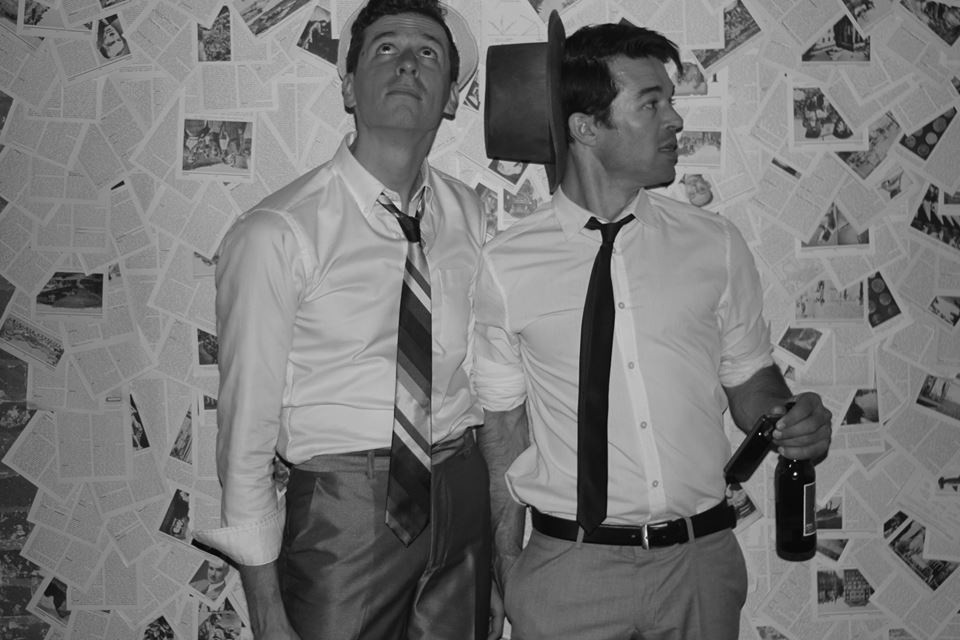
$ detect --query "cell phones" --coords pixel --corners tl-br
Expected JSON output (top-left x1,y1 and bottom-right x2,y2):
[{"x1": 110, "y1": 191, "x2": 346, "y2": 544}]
[{"x1": 722, "y1": 413, "x2": 786, "y2": 482}]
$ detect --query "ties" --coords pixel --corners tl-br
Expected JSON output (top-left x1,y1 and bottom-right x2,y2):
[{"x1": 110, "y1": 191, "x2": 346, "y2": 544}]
[
  {"x1": 377, "y1": 191, "x2": 431, "y2": 548},
  {"x1": 576, "y1": 213, "x2": 635, "y2": 533}
]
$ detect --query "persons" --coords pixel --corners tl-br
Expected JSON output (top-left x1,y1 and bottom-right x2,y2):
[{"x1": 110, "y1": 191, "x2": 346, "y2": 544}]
[
  {"x1": 471, "y1": 21, "x2": 834, "y2": 640},
  {"x1": 200, "y1": 0, "x2": 508, "y2": 640}
]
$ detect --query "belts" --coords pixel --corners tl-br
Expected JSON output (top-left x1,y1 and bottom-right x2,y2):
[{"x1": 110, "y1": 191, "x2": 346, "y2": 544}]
[{"x1": 534, "y1": 496, "x2": 739, "y2": 549}]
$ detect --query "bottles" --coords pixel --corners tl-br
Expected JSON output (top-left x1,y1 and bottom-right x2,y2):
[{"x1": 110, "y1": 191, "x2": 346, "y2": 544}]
[{"x1": 775, "y1": 402, "x2": 817, "y2": 562}]
